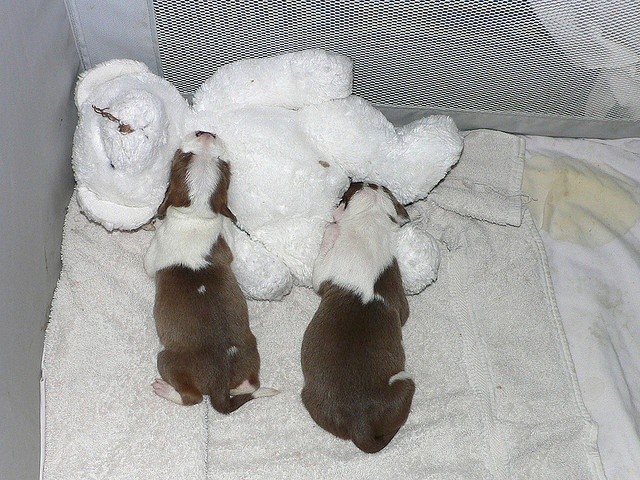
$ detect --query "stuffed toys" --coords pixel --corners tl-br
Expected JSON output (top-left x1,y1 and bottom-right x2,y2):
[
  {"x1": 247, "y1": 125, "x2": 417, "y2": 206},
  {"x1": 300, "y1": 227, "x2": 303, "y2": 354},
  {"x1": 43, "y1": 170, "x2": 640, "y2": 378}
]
[{"x1": 71, "y1": 48, "x2": 464, "y2": 300}]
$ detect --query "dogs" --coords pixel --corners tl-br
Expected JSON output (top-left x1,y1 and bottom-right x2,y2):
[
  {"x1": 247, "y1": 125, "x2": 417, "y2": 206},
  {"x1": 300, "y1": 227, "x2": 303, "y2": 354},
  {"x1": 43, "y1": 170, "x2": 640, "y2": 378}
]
[
  {"x1": 143, "y1": 131, "x2": 282, "y2": 415},
  {"x1": 300, "y1": 182, "x2": 416, "y2": 454}
]
